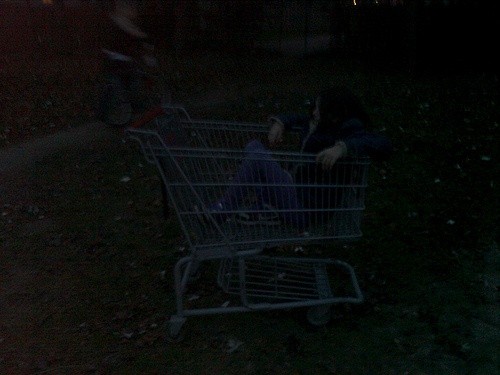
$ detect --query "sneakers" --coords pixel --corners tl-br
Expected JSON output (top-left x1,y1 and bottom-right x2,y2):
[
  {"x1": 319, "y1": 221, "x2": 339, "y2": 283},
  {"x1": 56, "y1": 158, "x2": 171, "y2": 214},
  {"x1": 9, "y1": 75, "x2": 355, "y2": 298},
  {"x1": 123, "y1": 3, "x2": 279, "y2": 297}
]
[
  {"x1": 195, "y1": 206, "x2": 218, "y2": 236},
  {"x1": 237, "y1": 209, "x2": 281, "y2": 226}
]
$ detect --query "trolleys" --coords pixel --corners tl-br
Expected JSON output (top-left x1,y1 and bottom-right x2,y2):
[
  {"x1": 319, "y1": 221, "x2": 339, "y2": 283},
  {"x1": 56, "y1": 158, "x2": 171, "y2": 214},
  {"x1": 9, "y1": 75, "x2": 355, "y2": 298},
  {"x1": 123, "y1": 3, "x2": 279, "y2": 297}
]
[{"x1": 123, "y1": 105, "x2": 373, "y2": 343}]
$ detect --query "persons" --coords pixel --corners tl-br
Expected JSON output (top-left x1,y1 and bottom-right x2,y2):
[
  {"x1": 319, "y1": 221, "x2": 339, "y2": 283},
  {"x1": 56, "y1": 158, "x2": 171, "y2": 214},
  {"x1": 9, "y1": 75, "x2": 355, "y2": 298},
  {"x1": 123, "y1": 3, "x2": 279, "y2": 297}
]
[
  {"x1": 189, "y1": 89, "x2": 393, "y2": 231},
  {"x1": 100, "y1": 39, "x2": 161, "y2": 121}
]
